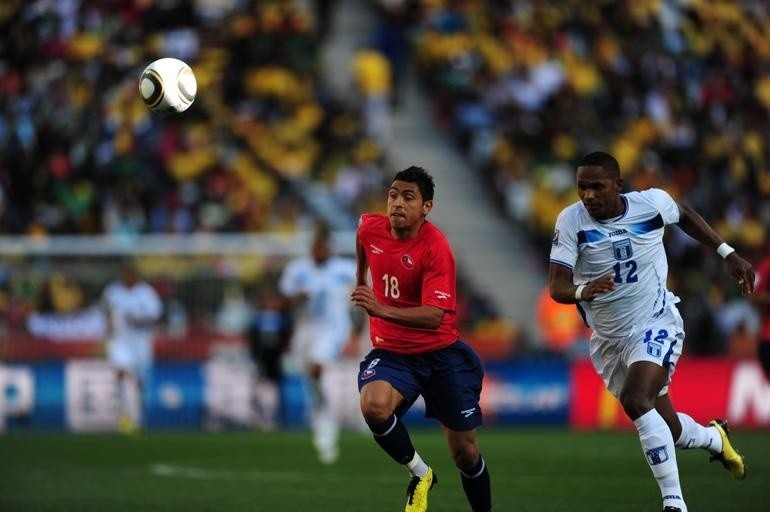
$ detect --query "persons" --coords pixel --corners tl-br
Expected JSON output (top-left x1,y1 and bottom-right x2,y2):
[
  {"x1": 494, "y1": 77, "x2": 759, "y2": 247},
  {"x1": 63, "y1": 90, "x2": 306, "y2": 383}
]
[
  {"x1": 0, "y1": 0, "x2": 769, "y2": 431},
  {"x1": 348, "y1": 164, "x2": 494, "y2": 512},
  {"x1": 263, "y1": 217, "x2": 366, "y2": 465},
  {"x1": 546, "y1": 151, "x2": 761, "y2": 511}
]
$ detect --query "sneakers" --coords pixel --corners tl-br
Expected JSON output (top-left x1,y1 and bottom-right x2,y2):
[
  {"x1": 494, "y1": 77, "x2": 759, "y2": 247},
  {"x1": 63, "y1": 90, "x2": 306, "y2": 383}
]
[
  {"x1": 309, "y1": 411, "x2": 343, "y2": 468},
  {"x1": 405, "y1": 460, "x2": 438, "y2": 512},
  {"x1": 707, "y1": 418, "x2": 747, "y2": 481}
]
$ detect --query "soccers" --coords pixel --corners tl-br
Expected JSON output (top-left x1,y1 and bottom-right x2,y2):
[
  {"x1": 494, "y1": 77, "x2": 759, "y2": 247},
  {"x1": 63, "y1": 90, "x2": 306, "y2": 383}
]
[{"x1": 139, "y1": 58, "x2": 197, "y2": 114}]
